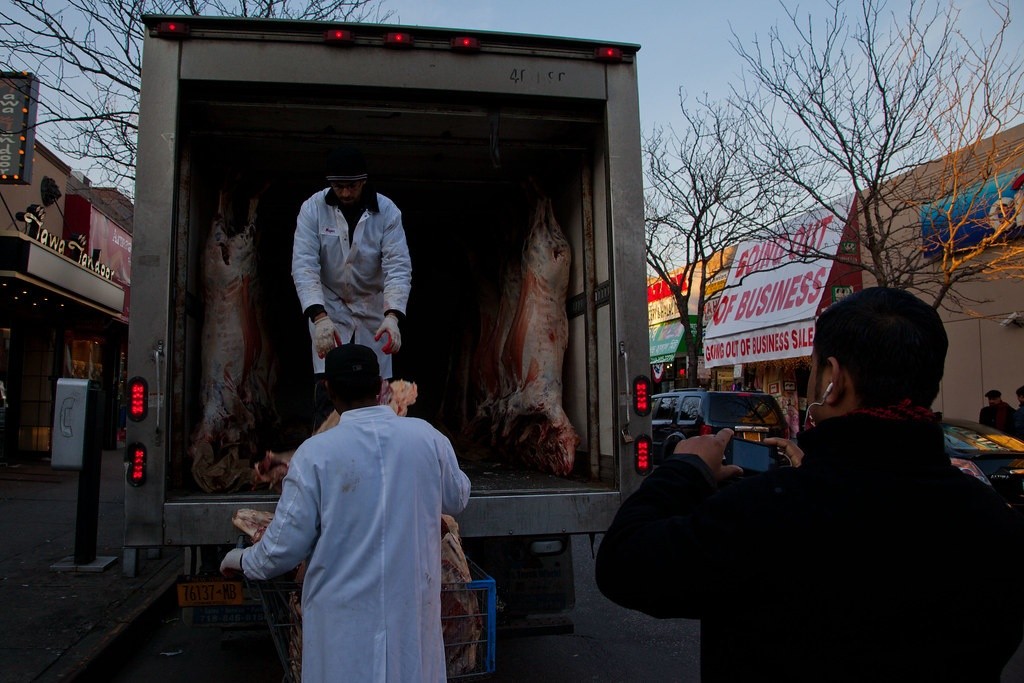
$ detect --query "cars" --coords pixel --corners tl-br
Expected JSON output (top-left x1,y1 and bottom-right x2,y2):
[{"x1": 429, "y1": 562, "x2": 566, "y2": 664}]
[{"x1": 939, "y1": 419, "x2": 1023, "y2": 505}]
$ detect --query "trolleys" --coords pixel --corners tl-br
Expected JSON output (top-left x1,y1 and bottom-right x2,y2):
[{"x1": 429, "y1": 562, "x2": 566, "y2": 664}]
[{"x1": 235, "y1": 536, "x2": 498, "y2": 683}]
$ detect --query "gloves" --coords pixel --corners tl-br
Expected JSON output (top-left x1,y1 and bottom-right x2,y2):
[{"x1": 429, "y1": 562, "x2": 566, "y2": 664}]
[
  {"x1": 313, "y1": 316, "x2": 342, "y2": 359},
  {"x1": 376, "y1": 315, "x2": 401, "y2": 354},
  {"x1": 220, "y1": 548, "x2": 245, "y2": 578}
]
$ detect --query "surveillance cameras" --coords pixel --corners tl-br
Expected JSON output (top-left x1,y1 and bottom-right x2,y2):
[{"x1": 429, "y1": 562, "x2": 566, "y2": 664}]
[{"x1": 999, "y1": 312, "x2": 1021, "y2": 328}]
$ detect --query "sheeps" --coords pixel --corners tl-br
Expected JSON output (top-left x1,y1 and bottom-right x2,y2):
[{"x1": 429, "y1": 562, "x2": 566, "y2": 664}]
[
  {"x1": 436, "y1": 177, "x2": 582, "y2": 477},
  {"x1": 187, "y1": 171, "x2": 296, "y2": 494},
  {"x1": 313, "y1": 380, "x2": 419, "y2": 433},
  {"x1": 232, "y1": 508, "x2": 484, "y2": 683}
]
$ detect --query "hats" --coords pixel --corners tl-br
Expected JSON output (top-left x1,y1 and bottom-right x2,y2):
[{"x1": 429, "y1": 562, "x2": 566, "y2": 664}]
[
  {"x1": 323, "y1": 149, "x2": 367, "y2": 181},
  {"x1": 985, "y1": 389, "x2": 1001, "y2": 397}
]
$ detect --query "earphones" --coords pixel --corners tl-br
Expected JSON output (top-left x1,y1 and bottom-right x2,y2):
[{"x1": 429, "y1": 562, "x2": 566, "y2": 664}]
[{"x1": 823, "y1": 383, "x2": 834, "y2": 399}]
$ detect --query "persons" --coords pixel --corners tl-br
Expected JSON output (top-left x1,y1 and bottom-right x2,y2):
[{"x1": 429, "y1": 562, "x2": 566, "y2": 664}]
[
  {"x1": 219, "y1": 344, "x2": 472, "y2": 683},
  {"x1": 979, "y1": 385, "x2": 1024, "y2": 440},
  {"x1": 595, "y1": 287, "x2": 1024, "y2": 683},
  {"x1": 291, "y1": 149, "x2": 413, "y2": 434}
]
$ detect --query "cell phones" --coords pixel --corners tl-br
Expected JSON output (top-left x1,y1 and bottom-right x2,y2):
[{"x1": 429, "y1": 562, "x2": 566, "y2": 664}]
[{"x1": 726, "y1": 435, "x2": 779, "y2": 474}]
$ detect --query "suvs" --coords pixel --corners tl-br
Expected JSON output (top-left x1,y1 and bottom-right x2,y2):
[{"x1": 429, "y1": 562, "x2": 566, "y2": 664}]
[{"x1": 651, "y1": 387, "x2": 791, "y2": 465}]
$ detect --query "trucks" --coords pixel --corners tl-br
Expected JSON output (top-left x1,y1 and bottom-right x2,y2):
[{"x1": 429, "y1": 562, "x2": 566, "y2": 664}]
[{"x1": 109, "y1": 12, "x2": 655, "y2": 632}]
